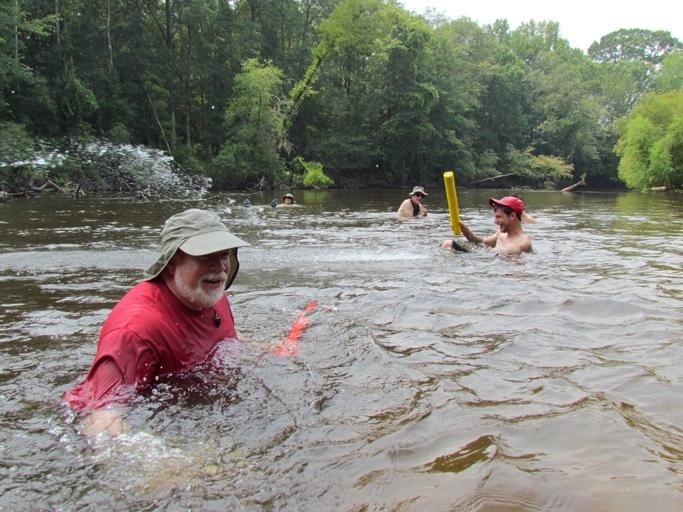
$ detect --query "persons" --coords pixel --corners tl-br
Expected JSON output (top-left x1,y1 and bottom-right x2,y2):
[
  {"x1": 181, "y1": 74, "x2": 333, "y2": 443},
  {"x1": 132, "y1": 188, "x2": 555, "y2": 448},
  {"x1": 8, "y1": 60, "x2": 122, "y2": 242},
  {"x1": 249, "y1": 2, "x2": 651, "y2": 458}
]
[
  {"x1": 511, "y1": 195, "x2": 536, "y2": 224},
  {"x1": 283, "y1": 192, "x2": 296, "y2": 204},
  {"x1": 441, "y1": 195, "x2": 533, "y2": 255},
  {"x1": 61, "y1": 208, "x2": 252, "y2": 432},
  {"x1": 397, "y1": 186, "x2": 429, "y2": 218}
]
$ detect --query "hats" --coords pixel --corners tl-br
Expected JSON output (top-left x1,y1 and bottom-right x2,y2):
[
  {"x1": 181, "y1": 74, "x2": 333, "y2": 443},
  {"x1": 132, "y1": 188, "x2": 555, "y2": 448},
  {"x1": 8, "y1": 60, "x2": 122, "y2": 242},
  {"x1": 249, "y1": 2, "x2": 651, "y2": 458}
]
[
  {"x1": 408, "y1": 185, "x2": 429, "y2": 197},
  {"x1": 282, "y1": 193, "x2": 296, "y2": 203},
  {"x1": 143, "y1": 208, "x2": 252, "y2": 291},
  {"x1": 489, "y1": 196, "x2": 524, "y2": 215}
]
